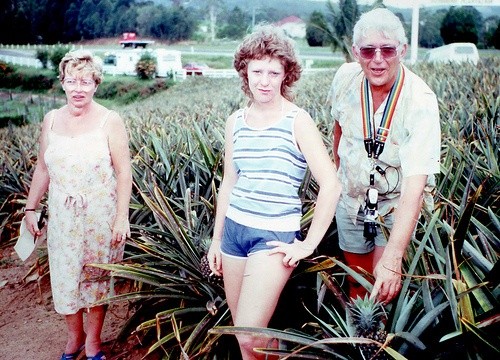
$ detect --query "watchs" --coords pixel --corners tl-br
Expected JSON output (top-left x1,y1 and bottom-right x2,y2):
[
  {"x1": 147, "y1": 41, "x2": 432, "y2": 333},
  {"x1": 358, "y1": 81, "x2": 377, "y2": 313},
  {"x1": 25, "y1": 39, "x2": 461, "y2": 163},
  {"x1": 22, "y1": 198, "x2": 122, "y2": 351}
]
[{"x1": 23, "y1": 207, "x2": 35, "y2": 212}]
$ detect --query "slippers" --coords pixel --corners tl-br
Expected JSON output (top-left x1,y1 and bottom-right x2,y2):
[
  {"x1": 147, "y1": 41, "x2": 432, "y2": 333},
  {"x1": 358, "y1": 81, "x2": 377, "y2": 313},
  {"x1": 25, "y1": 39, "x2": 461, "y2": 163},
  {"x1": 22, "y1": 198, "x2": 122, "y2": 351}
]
[
  {"x1": 60, "y1": 343, "x2": 86, "y2": 360},
  {"x1": 86, "y1": 351, "x2": 107, "y2": 360}
]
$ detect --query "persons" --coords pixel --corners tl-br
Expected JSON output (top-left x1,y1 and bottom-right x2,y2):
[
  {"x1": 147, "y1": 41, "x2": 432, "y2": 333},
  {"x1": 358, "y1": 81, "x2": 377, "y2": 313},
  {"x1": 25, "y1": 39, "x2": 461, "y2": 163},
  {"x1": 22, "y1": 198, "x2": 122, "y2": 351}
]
[
  {"x1": 331, "y1": 9, "x2": 441, "y2": 345},
  {"x1": 207, "y1": 31, "x2": 343, "y2": 360},
  {"x1": 25, "y1": 50, "x2": 132, "y2": 360}
]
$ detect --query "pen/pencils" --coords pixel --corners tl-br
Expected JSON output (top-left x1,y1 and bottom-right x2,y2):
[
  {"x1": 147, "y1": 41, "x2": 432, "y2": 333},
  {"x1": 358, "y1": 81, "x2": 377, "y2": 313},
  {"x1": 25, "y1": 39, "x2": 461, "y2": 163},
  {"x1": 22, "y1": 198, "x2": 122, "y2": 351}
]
[{"x1": 34, "y1": 205, "x2": 46, "y2": 243}]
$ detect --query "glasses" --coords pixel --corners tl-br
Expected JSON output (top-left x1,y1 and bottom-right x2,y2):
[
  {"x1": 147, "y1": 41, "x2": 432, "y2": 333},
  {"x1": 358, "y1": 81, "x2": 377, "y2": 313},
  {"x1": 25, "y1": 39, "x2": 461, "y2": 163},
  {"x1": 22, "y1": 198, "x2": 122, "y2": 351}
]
[
  {"x1": 62, "y1": 78, "x2": 96, "y2": 87},
  {"x1": 360, "y1": 46, "x2": 399, "y2": 60}
]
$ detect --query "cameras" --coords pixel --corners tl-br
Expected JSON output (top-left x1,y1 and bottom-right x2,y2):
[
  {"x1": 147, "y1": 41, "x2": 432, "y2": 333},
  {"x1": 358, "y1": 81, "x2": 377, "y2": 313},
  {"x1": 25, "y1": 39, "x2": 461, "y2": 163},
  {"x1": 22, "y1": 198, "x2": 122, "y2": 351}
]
[{"x1": 363, "y1": 188, "x2": 380, "y2": 238}]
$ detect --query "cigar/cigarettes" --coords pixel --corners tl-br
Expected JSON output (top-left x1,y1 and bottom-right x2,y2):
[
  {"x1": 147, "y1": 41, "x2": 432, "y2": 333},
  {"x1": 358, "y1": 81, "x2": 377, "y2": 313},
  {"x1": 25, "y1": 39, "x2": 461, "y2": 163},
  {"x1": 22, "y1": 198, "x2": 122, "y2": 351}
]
[{"x1": 209, "y1": 269, "x2": 216, "y2": 276}]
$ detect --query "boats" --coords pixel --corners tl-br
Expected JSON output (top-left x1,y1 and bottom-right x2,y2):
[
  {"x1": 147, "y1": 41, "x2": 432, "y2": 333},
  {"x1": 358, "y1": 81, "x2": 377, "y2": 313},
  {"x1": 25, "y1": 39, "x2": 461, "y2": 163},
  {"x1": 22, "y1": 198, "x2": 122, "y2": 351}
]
[{"x1": 68, "y1": 32, "x2": 214, "y2": 79}]
